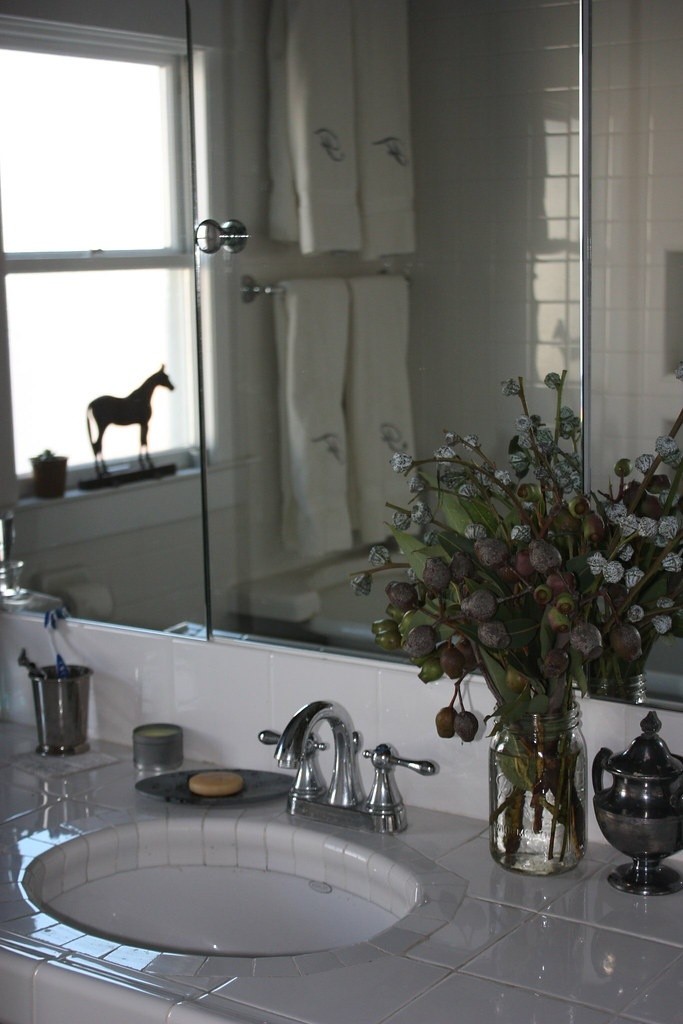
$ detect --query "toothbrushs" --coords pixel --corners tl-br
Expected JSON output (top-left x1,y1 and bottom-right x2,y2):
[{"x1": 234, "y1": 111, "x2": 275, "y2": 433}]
[
  {"x1": 42, "y1": 612, "x2": 69, "y2": 675},
  {"x1": 57, "y1": 607, "x2": 68, "y2": 619}
]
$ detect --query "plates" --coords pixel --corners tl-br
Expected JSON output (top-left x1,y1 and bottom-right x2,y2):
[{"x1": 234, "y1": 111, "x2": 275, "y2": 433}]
[{"x1": 134, "y1": 769, "x2": 299, "y2": 805}]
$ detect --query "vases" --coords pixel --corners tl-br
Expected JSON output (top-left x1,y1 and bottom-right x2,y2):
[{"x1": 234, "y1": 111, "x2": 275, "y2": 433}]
[{"x1": 490, "y1": 702, "x2": 588, "y2": 877}]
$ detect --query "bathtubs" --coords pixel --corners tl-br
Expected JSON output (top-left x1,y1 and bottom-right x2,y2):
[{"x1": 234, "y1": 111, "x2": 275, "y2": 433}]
[{"x1": 241, "y1": 540, "x2": 681, "y2": 702}]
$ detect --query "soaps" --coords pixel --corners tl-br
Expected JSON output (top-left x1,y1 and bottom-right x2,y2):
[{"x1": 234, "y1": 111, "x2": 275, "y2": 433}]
[{"x1": 190, "y1": 770, "x2": 246, "y2": 797}]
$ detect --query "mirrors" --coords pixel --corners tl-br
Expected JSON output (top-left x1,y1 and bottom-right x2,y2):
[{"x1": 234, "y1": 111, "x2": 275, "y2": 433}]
[{"x1": 0, "y1": 0, "x2": 683, "y2": 714}]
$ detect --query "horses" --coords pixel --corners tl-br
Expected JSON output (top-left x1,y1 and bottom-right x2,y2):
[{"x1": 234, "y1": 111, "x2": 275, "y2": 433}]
[{"x1": 86, "y1": 364, "x2": 174, "y2": 468}]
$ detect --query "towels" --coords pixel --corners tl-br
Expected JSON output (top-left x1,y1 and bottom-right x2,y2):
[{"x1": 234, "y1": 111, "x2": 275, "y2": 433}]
[
  {"x1": 268, "y1": 0, "x2": 363, "y2": 255},
  {"x1": 351, "y1": 0, "x2": 419, "y2": 261},
  {"x1": 347, "y1": 274, "x2": 428, "y2": 551},
  {"x1": 273, "y1": 276, "x2": 356, "y2": 567}
]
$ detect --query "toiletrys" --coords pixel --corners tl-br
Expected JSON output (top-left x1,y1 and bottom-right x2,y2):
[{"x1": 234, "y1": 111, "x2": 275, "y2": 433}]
[{"x1": 17, "y1": 647, "x2": 49, "y2": 679}]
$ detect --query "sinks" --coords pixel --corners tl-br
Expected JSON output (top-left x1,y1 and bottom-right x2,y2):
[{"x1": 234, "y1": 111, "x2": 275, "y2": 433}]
[{"x1": 20, "y1": 821, "x2": 422, "y2": 957}]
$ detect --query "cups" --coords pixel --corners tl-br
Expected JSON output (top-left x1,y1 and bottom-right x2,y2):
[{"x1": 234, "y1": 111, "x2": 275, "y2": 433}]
[
  {"x1": 30, "y1": 449, "x2": 69, "y2": 499},
  {"x1": 28, "y1": 664, "x2": 94, "y2": 757}
]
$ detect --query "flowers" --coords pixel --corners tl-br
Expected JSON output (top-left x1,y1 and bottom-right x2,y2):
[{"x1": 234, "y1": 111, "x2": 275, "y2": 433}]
[{"x1": 349, "y1": 354, "x2": 683, "y2": 748}]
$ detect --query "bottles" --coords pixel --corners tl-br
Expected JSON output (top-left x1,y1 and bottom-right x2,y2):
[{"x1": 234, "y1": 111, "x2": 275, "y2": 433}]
[{"x1": 132, "y1": 723, "x2": 184, "y2": 773}]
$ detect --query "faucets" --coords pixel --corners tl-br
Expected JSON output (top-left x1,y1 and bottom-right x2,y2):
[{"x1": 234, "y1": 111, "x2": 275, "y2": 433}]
[{"x1": 271, "y1": 695, "x2": 365, "y2": 807}]
